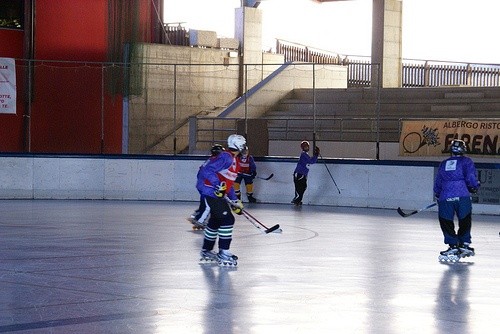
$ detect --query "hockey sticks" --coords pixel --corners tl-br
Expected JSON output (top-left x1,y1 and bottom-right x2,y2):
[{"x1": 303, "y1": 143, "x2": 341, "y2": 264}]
[
  {"x1": 242, "y1": 212, "x2": 283, "y2": 233},
  {"x1": 397, "y1": 181, "x2": 480, "y2": 218},
  {"x1": 238, "y1": 172, "x2": 274, "y2": 180},
  {"x1": 243, "y1": 209, "x2": 280, "y2": 234},
  {"x1": 316, "y1": 145, "x2": 341, "y2": 194}
]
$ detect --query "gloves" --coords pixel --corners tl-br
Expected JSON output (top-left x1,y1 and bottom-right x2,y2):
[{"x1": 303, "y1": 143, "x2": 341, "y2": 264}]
[
  {"x1": 231, "y1": 200, "x2": 243, "y2": 214},
  {"x1": 468, "y1": 187, "x2": 477, "y2": 194},
  {"x1": 314, "y1": 146, "x2": 320, "y2": 155},
  {"x1": 251, "y1": 172, "x2": 256, "y2": 178},
  {"x1": 213, "y1": 181, "x2": 226, "y2": 198}
]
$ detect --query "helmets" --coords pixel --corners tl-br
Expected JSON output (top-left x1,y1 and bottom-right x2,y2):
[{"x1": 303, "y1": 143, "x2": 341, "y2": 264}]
[
  {"x1": 211, "y1": 145, "x2": 223, "y2": 155},
  {"x1": 228, "y1": 134, "x2": 246, "y2": 151},
  {"x1": 301, "y1": 142, "x2": 307, "y2": 149},
  {"x1": 242, "y1": 146, "x2": 249, "y2": 152},
  {"x1": 451, "y1": 139, "x2": 465, "y2": 154}
]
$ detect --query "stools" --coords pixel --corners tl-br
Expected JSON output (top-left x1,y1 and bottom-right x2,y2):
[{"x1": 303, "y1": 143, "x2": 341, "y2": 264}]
[{"x1": 190, "y1": 29, "x2": 238, "y2": 50}]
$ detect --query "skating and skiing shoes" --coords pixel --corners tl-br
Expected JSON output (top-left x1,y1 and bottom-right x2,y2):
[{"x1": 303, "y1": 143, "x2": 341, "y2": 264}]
[
  {"x1": 458, "y1": 241, "x2": 474, "y2": 258},
  {"x1": 439, "y1": 245, "x2": 460, "y2": 261},
  {"x1": 192, "y1": 221, "x2": 208, "y2": 229},
  {"x1": 186, "y1": 214, "x2": 197, "y2": 224},
  {"x1": 217, "y1": 251, "x2": 238, "y2": 266},
  {"x1": 200, "y1": 250, "x2": 218, "y2": 261},
  {"x1": 291, "y1": 200, "x2": 302, "y2": 207}
]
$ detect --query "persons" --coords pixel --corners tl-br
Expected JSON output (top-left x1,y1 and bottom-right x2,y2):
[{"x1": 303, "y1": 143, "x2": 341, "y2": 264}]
[
  {"x1": 291, "y1": 142, "x2": 320, "y2": 206},
  {"x1": 187, "y1": 144, "x2": 227, "y2": 229},
  {"x1": 197, "y1": 134, "x2": 246, "y2": 266},
  {"x1": 433, "y1": 139, "x2": 479, "y2": 262},
  {"x1": 232, "y1": 146, "x2": 257, "y2": 204}
]
